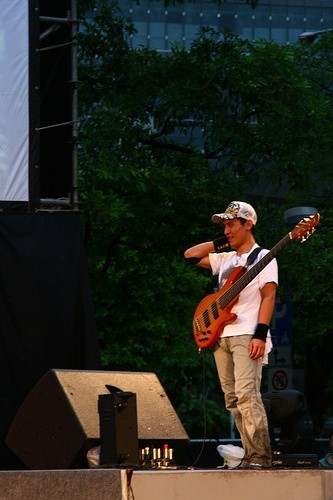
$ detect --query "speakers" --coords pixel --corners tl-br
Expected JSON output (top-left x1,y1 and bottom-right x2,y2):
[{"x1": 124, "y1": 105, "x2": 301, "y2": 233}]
[{"x1": 4, "y1": 369, "x2": 190, "y2": 470}]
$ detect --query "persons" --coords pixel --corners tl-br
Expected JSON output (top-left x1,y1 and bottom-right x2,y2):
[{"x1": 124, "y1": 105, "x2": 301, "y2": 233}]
[
  {"x1": 183, "y1": 201, "x2": 278, "y2": 470},
  {"x1": 318, "y1": 430, "x2": 333, "y2": 469}
]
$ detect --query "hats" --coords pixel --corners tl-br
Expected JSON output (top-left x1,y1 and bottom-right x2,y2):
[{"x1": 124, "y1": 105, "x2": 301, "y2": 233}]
[{"x1": 211, "y1": 201, "x2": 257, "y2": 225}]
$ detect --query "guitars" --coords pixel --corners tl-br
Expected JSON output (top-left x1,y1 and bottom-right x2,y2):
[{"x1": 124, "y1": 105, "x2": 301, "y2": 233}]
[{"x1": 192, "y1": 212, "x2": 321, "y2": 349}]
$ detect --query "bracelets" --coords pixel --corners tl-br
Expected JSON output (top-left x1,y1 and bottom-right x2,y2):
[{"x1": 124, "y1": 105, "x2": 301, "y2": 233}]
[
  {"x1": 213, "y1": 236, "x2": 230, "y2": 251},
  {"x1": 252, "y1": 323, "x2": 269, "y2": 343}
]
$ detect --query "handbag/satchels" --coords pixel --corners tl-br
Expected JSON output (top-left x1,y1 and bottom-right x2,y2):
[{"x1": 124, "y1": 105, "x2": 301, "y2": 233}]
[{"x1": 217, "y1": 444, "x2": 244, "y2": 468}]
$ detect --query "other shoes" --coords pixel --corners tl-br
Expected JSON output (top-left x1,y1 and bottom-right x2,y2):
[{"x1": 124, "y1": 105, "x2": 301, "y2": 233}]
[{"x1": 231, "y1": 458, "x2": 272, "y2": 470}]
